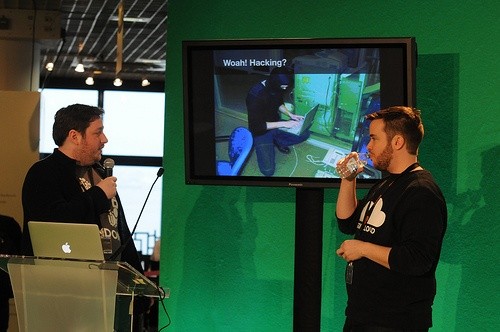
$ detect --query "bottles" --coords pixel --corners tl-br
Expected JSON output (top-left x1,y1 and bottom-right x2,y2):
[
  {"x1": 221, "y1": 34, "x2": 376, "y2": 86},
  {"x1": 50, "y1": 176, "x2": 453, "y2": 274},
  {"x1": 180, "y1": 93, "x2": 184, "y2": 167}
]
[{"x1": 335, "y1": 151, "x2": 371, "y2": 179}]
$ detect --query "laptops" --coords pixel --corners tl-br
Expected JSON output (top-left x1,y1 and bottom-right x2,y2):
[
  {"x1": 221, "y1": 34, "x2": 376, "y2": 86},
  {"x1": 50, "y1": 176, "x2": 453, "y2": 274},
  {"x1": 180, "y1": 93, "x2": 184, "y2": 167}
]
[
  {"x1": 26, "y1": 222, "x2": 106, "y2": 262},
  {"x1": 279, "y1": 104, "x2": 320, "y2": 136}
]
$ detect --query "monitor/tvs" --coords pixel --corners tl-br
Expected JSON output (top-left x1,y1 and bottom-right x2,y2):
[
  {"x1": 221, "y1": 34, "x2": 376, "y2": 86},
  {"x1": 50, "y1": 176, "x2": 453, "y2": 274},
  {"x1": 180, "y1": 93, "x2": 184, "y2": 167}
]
[{"x1": 181, "y1": 37, "x2": 417, "y2": 189}]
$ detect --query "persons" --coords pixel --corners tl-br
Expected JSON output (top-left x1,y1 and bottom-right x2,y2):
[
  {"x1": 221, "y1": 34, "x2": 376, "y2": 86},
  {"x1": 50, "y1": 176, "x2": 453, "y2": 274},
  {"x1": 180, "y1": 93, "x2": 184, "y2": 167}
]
[
  {"x1": 0, "y1": 103, "x2": 153, "y2": 332},
  {"x1": 245, "y1": 66, "x2": 305, "y2": 176},
  {"x1": 335, "y1": 105, "x2": 447, "y2": 332}
]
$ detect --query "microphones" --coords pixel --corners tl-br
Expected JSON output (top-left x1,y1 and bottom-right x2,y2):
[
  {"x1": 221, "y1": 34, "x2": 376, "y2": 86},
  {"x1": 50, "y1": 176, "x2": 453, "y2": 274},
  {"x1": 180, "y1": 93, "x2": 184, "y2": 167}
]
[
  {"x1": 104, "y1": 159, "x2": 114, "y2": 177},
  {"x1": 108, "y1": 168, "x2": 165, "y2": 263}
]
